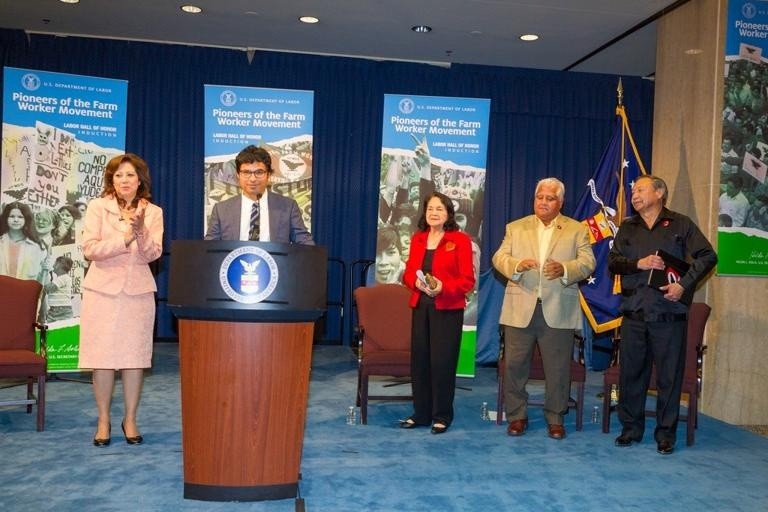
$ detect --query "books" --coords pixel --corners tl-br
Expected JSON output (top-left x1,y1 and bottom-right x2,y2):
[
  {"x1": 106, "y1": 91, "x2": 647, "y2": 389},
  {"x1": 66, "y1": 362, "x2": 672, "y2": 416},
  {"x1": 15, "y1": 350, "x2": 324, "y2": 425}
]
[{"x1": 647, "y1": 249, "x2": 697, "y2": 308}]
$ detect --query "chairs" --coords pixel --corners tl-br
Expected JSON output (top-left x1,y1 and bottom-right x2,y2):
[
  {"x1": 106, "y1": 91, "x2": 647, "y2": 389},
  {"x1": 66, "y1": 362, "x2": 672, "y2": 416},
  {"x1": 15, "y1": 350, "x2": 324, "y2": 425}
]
[
  {"x1": 603, "y1": 299, "x2": 711, "y2": 446},
  {"x1": 1, "y1": 276, "x2": 49, "y2": 430},
  {"x1": 351, "y1": 285, "x2": 419, "y2": 424},
  {"x1": 498, "y1": 324, "x2": 586, "y2": 431}
]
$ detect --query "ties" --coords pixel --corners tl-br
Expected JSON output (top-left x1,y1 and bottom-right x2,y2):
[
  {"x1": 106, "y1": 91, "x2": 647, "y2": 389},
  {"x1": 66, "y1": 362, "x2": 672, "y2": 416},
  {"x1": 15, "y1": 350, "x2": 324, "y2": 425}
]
[{"x1": 249, "y1": 203, "x2": 260, "y2": 241}]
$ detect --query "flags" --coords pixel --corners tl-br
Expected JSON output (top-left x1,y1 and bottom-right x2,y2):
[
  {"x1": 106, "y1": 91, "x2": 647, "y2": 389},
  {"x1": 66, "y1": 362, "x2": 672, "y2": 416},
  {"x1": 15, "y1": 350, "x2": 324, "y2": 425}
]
[{"x1": 570, "y1": 106, "x2": 647, "y2": 337}]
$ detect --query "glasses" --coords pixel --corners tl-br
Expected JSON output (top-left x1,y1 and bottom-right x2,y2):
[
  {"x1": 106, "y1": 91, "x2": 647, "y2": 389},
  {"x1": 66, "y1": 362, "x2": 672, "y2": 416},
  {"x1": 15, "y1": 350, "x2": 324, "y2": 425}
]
[{"x1": 238, "y1": 169, "x2": 269, "y2": 178}]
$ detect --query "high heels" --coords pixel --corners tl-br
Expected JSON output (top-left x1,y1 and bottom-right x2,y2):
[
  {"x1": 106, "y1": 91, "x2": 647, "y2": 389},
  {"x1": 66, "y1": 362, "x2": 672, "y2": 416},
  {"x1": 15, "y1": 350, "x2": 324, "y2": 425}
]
[
  {"x1": 122, "y1": 421, "x2": 142, "y2": 445},
  {"x1": 94, "y1": 423, "x2": 111, "y2": 448}
]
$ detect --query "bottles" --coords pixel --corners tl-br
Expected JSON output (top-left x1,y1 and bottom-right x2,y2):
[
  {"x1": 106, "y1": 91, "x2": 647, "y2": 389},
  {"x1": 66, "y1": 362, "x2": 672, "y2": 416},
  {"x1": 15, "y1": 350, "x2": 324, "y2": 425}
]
[
  {"x1": 480, "y1": 402, "x2": 488, "y2": 420},
  {"x1": 346, "y1": 406, "x2": 356, "y2": 426},
  {"x1": 591, "y1": 406, "x2": 600, "y2": 423}
]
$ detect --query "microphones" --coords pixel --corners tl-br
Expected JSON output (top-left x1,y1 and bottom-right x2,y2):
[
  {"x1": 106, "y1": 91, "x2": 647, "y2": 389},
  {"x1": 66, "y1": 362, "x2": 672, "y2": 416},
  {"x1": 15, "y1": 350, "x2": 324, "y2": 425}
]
[{"x1": 256, "y1": 192, "x2": 263, "y2": 241}]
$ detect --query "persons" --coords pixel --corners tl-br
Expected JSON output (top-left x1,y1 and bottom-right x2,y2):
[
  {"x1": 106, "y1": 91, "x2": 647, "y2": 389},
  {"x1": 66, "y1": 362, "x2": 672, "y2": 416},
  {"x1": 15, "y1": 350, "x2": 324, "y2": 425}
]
[
  {"x1": 0, "y1": 201, "x2": 86, "y2": 323},
  {"x1": 491, "y1": 177, "x2": 597, "y2": 438},
  {"x1": 204, "y1": 145, "x2": 315, "y2": 247},
  {"x1": 718, "y1": 58, "x2": 768, "y2": 233},
  {"x1": 401, "y1": 192, "x2": 476, "y2": 435},
  {"x1": 77, "y1": 154, "x2": 164, "y2": 448},
  {"x1": 607, "y1": 175, "x2": 718, "y2": 455},
  {"x1": 375, "y1": 134, "x2": 483, "y2": 285}
]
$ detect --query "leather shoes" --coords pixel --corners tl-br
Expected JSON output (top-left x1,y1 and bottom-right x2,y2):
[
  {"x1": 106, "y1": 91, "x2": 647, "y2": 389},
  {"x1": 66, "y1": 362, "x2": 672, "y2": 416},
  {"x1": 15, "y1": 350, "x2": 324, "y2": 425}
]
[
  {"x1": 400, "y1": 416, "x2": 432, "y2": 428},
  {"x1": 548, "y1": 424, "x2": 563, "y2": 438},
  {"x1": 616, "y1": 435, "x2": 636, "y2": 446},
  {"x1": 658, "y1": 441, "x2": 674, "y2": 454},
  {"x1": 431, "y1": 420, "x2": 449, "y2": 434},
  {"x1": 508, "y1": 417, "x2": 528, "y2": 436}
]
